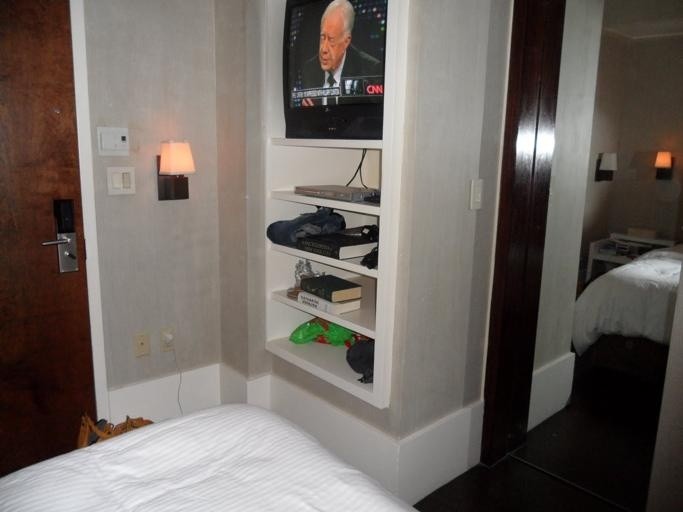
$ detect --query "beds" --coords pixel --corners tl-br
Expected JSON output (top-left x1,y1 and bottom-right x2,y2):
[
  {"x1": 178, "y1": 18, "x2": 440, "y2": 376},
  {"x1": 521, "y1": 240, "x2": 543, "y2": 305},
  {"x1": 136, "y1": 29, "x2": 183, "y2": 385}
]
[
  {"x1": 0, "y1": 402, "x2": 418, "y2": 512},
  {"x1": 570, "y1": 243, "x2": 683, "y2": 379}
]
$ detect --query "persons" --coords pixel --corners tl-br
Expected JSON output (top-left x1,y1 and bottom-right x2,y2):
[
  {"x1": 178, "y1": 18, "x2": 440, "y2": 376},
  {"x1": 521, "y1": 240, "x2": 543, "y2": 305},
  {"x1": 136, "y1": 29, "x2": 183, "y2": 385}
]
[{"x1": 288, "y1": 1, "x2": 380, "y2": 107}]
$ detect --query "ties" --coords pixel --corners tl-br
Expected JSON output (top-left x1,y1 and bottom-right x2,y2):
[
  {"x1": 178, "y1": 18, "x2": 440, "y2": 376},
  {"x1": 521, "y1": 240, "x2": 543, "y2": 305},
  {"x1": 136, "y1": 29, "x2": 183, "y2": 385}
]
[{"x1": 327, "y1": 76, "x2": 337, "y2": 106}]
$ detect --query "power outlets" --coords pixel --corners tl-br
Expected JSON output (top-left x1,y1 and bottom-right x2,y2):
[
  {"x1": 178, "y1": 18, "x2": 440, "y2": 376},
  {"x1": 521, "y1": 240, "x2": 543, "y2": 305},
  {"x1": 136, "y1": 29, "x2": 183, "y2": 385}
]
[{"x1": 160, "y1": 326, "x2": 176, "y2": 351}]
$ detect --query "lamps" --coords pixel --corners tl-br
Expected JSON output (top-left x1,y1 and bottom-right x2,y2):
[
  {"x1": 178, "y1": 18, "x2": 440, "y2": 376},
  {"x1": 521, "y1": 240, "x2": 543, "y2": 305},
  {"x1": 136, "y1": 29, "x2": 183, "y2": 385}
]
[
  {"x1": 595, "y1": 151, "x2": 620, "y2": 182},
  {"x1": 654, "y1": 150, "x2": 675, "y2": 179},
  {"x1": 155, "y1": 137, "x2": 198, "y2": 203}
]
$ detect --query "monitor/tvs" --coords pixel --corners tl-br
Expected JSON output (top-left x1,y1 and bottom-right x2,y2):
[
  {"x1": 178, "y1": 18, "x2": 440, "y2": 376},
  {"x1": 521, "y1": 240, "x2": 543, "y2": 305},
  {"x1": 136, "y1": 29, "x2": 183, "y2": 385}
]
[{"x1": 283, "y1": 0, "x2": 388, "y2": 139}]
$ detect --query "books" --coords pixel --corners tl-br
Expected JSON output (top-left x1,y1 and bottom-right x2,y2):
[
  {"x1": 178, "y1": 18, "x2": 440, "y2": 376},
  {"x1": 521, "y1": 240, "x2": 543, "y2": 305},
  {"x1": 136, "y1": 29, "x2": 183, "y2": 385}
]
[
  {"x1": 297, "y1": 234, "x2": 377, "y2": 259},
  {"x1": 299, "y1": 275, "x2": 362, "y2": 301},
  {"x1": 296, "y1": 291, "x2": 361, "y2": 315}
]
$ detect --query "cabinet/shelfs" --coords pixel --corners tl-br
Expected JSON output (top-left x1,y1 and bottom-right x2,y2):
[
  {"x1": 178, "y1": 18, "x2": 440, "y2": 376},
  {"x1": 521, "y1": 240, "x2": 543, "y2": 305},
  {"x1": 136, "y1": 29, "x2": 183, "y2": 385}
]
[
  {"x1": 266, "y1": 135, "x2": 384, "y2": 413},
  {"x1": 584, "y1": 235, "x2": 675, "y2": 285}
]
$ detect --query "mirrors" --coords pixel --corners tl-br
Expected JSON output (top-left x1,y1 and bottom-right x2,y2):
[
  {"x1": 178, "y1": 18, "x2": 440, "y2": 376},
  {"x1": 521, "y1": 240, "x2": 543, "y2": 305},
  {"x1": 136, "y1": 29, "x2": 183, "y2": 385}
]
[{"x1": 509, "y1": 0, "x2": 682, "y2": 512}]
